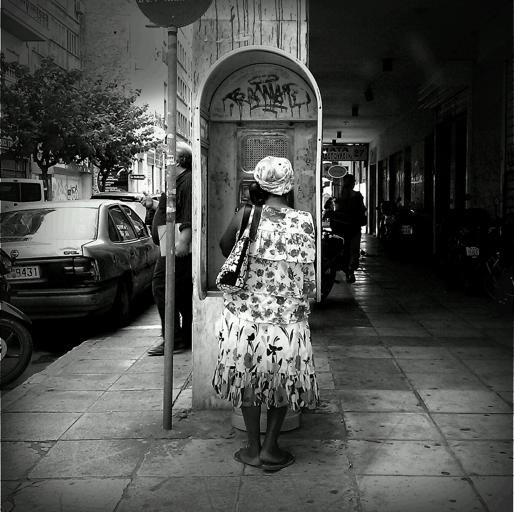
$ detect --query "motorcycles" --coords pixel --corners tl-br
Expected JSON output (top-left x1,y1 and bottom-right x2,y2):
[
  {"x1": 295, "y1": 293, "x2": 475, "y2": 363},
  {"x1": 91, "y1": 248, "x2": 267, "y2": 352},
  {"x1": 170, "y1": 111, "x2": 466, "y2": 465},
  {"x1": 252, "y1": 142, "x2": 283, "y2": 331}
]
[
  {"x1": 376, "y1": 197, "x2": 417, "y2": 259},
  {"x1": 439, "y1": 194, "x2": 514, "y2": 305},
  {"x1": 0, "y1": 248, "x2": 34, "y2": 387},
  {"x1": 321, "y1": 234, "x2": 352, "y2": 298}
]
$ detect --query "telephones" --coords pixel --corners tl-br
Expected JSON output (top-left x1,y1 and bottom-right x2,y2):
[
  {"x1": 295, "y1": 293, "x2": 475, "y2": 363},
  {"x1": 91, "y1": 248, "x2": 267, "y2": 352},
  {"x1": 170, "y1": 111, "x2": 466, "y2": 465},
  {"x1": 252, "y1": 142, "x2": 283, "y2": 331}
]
[{"x1": 249, "y1": 182, "x2": 261, "y2": 205}]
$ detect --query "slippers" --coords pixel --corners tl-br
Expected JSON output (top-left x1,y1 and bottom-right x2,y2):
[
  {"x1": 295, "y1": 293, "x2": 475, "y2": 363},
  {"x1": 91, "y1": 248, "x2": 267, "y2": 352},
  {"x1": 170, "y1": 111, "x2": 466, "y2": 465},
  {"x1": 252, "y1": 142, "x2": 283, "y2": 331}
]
[
  {"x1": 262, "y1": 450, "x2": 296, "y2": 471},
  {"x1": 233, "y1": 447, "x2": 263, "y2": 468}
]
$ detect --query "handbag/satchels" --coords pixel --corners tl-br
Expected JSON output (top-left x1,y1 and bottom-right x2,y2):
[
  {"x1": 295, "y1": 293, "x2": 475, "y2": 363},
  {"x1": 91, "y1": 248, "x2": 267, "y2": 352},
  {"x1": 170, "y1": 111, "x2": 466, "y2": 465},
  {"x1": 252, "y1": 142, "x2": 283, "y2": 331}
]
[{"x1": 215, "y1": 235, "x2": 253, "y2": 294}]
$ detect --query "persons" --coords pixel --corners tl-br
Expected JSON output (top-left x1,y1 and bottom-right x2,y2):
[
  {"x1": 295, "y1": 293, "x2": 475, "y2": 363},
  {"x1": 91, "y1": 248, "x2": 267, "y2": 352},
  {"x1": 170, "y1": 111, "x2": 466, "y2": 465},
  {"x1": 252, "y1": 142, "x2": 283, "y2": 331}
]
[
  {"x1": 135, "y1": 142, "x2": 192, "y2": 354},
  {"x1": 324, "y1": 196, "x2": 336, "y2": 219},
  {"x1": 219, "y1": 156, "x2": 317, "y2": 473},
  {"x1": 332, "y1": 172, "x2": 366, "y2": 280}
]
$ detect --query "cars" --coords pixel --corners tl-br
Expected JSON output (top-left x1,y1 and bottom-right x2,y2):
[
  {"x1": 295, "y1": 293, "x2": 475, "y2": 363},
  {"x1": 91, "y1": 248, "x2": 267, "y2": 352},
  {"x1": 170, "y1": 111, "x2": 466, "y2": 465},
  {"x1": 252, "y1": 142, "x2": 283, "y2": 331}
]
[{"x1": 0, "y1": 178, "x2": 162, "y2": 320}]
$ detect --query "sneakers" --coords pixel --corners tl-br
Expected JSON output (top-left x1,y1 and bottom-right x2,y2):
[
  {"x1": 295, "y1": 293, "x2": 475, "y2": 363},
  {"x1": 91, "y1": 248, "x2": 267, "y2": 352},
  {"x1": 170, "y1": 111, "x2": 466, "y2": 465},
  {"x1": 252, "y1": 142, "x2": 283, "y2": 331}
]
[{"x1": 147, "y1": 340, "x2": 185, "y2": 356}]
